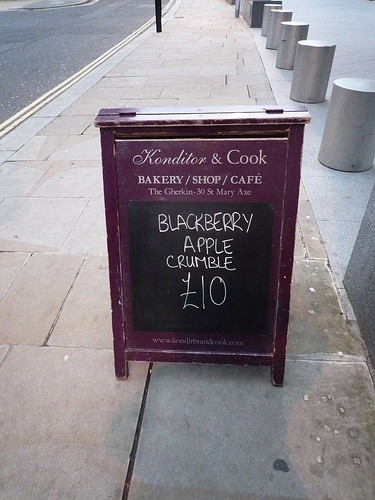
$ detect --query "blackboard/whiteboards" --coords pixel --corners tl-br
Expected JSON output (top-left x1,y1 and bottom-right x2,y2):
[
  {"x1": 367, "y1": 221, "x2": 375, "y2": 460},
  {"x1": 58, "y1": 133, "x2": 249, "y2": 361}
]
[{"x1": 93, "y1": 110, "x2": 312, "y2": 368}]
